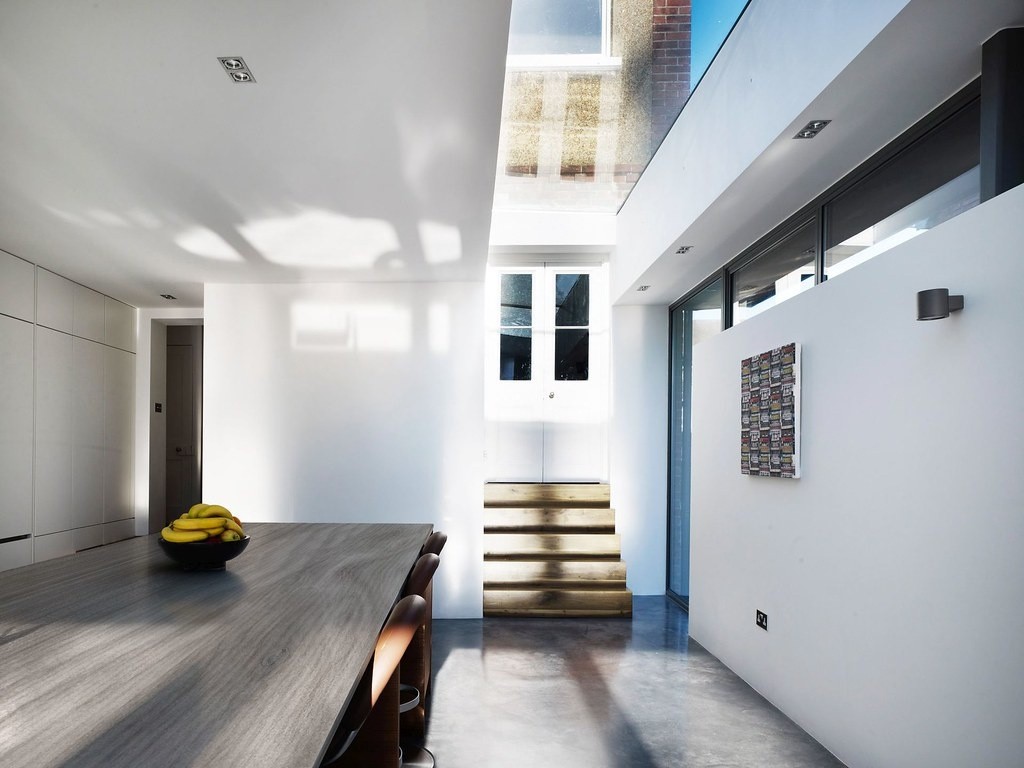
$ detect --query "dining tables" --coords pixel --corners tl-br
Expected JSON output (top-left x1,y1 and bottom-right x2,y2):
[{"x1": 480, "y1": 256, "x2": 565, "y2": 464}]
[{"x1": 1, "y1": 522, "x2": 434, "y2": 768}]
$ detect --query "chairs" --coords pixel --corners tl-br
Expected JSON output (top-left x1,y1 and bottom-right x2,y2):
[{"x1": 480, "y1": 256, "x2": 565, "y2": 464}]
[
  {"x1": 402, "y1": 552, "x2": 440, "y2": 596},
  {"x1": 316, "y1": 594, "x2": 426, "y2": 768},
  {"x1": 420, "y1": 531, "x2": 447, "y2": 557}
]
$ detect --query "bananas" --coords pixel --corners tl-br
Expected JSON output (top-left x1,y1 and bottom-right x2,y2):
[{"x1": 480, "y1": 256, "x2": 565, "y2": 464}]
[{"x1": 162, "y1": 504, "x2": 246, "y2": 544}]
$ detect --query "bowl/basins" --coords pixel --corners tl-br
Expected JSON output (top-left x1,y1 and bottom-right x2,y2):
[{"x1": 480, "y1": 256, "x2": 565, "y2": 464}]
[{"x1": 157, "y1": 536, "x2": 253, "y2": 571}]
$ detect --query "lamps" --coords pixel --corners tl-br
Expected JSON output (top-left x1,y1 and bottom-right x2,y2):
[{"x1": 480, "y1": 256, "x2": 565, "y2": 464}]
[{"x1": 916, "y1": 287, "x2": 965, "y2": 321}]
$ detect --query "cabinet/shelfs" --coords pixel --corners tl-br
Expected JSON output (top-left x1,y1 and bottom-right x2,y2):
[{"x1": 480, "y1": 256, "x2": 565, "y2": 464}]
[{"x1": 0, "y1": 249, "x2": 136, "y2": 572}]
